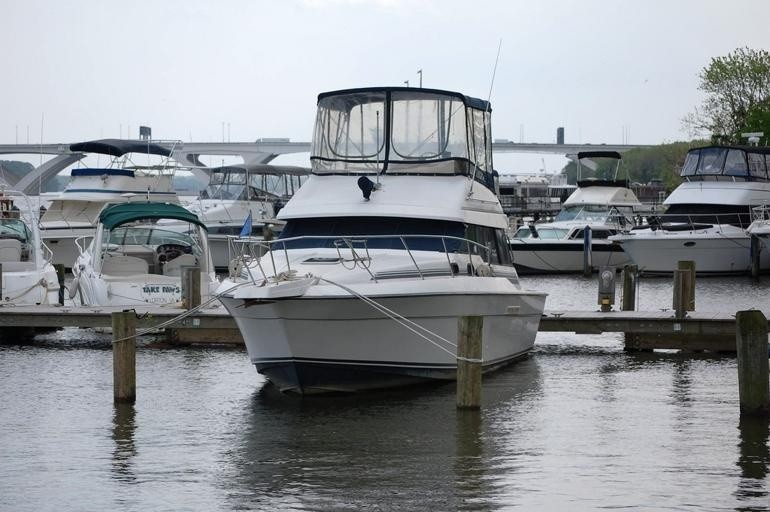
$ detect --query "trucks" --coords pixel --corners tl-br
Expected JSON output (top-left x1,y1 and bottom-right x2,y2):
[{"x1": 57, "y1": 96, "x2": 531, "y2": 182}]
[{"x1": 495, "y1": 138, "x2": 514, "y2": 144}]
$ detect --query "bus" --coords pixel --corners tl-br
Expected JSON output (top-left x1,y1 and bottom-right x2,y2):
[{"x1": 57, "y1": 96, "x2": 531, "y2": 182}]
[{"x1": 255, "y1": 137, "x2": 290, "y2": 142}]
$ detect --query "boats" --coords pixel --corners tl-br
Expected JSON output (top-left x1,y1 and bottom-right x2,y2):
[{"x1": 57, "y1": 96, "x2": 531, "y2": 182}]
[
  {"x1": 214, "y1": 70, "x2": 549, "y2": 400},
  {"x1": 0, "y1": 186, "x2": 58, "y2": 345},
  {"x1": 70, "y1": 205, "x2": 221, "y2": 336}
]
